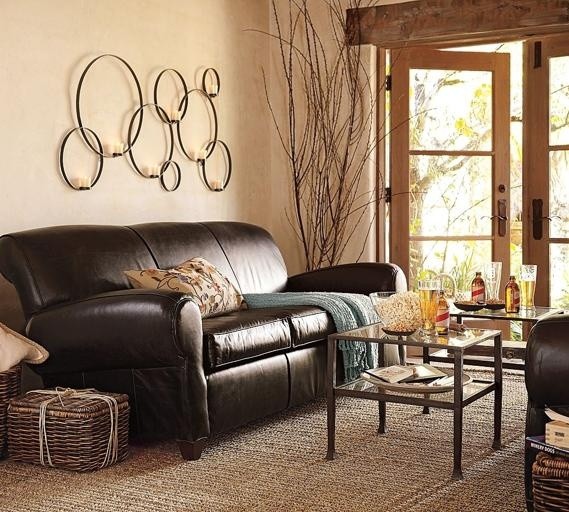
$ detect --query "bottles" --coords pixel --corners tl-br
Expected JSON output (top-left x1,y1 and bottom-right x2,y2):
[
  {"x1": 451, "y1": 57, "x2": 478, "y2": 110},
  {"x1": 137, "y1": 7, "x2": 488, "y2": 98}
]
[
  {"x1": 436, "y1": 291, "x2": 450, "y2": 335},
  {"x1": 471, "y1": 271, "x2": 485, "y2": 301},
  {"x1": 505, "y1": 276, "x2": 520, "y2": 313}
]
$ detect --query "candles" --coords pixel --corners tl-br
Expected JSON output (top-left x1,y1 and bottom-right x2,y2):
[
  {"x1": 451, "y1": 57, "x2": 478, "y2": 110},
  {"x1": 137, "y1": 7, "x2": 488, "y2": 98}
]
[
  {"x1": 215, "y1": 181, "x2": 223, "y2": 190},
  {"x1": 149, "y1": 165, "x2": 161, "y2": 177},
  {"x1": 80, "y1": 175, "x2": 92, "y2": 188},
  {"x1": 171, "y1": 111, "x2": 181, "y2": 121},
  {"x1": 209, "y1": 84, "x2": 219, "y2": 95},
  {"x1": 198, "y1": 147, "x2": 207, "y2": 160},
  {"x1": 114, "y1": 142, "x2": 124, "y2": 155}
]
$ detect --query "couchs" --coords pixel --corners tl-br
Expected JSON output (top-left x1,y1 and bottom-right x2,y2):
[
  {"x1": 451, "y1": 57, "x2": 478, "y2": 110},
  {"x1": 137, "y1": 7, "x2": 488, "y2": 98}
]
[
  {"x1": 524, "y1": 309, "x2": 569, "y2": 511},
  {"x1": 1, "y1": 221, "x2": 407, "y2": 461}
]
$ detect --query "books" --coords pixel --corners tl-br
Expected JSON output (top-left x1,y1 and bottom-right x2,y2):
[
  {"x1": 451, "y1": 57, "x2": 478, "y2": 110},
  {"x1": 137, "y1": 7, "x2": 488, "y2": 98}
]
[
  {"x1": 525, "y1": 436, "x2": 569, "y2": 456},
  {"x1": 373, "y1": 364, "x2": 436, "y2": 384}
]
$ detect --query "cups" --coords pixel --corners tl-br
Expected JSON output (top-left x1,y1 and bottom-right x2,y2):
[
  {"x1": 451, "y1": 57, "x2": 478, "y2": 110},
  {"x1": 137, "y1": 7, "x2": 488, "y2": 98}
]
[
  {"x1": 519, "y1": 265, "x2": 537, "y2": 310},
  {"x1": 418, "y1": 280, "x2": 438, "y2": 333},
  {"x1": 483, "y1": 262, "x2": 502, "y2": 299}
]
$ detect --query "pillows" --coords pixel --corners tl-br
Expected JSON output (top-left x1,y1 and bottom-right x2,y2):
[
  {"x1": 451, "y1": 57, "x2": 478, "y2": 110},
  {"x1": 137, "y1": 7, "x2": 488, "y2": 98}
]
[{"x1": 124, "y1": 257, "x2": 249, "y2": 319}]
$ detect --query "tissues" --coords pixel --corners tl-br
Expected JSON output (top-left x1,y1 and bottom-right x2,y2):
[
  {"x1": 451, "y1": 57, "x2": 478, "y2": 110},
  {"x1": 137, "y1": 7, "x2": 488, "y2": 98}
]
[{"x1": 544, "y1": 403, "x2": 569, "y2": 451}]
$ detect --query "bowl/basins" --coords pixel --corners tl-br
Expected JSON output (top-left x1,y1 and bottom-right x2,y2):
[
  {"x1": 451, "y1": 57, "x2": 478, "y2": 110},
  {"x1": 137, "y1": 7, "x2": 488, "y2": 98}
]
[{"x1": 370, "y1": 292, "x2": 419, "y2": 331}]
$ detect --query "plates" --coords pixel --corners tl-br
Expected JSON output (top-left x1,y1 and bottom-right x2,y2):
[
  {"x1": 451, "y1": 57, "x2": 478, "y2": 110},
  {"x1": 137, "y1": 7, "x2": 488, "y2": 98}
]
[
  {"x1": 360, "y1": 367, "x2": 472, "y2": 393},
  {"x1": 381, "y1": 328, "x2": 416, "y2": 336},
  {"x1": 455, "y1": 302, "x2": 487, "y2": 311},
  {"x1": 484, "y1": 303, "x2": 505, "y2": 310}
]
